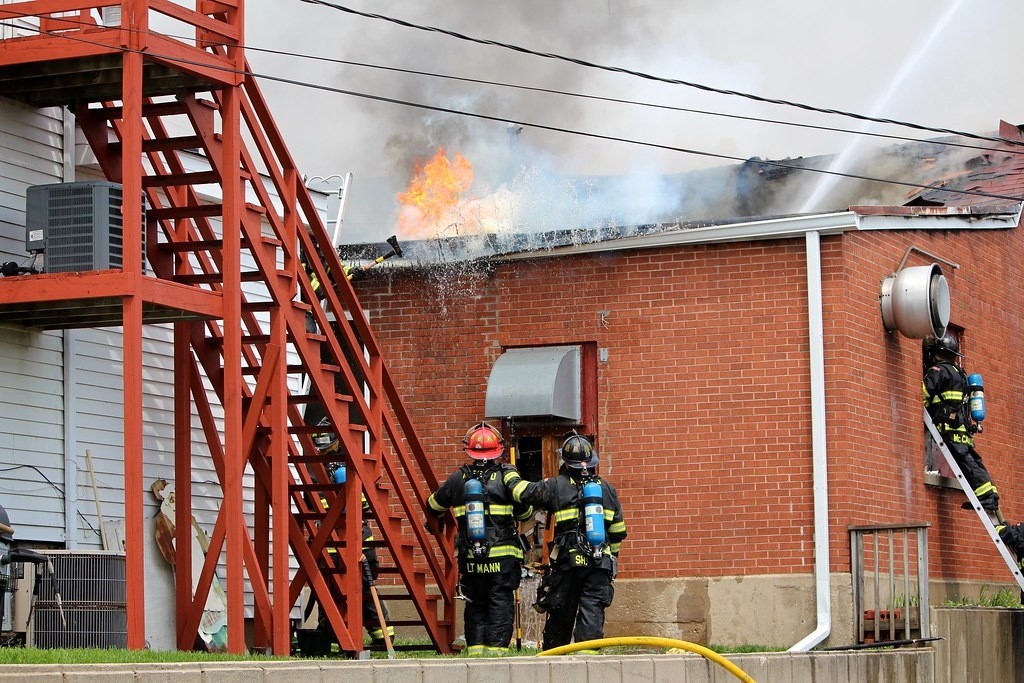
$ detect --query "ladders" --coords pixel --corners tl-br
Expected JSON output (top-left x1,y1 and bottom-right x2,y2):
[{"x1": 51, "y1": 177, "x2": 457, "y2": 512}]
[
  {"x1": 924, "y1": 408, "x2": 1024, "y2": 591},
  {"x1": 300, "y1": 172, "x2": 354, "y2": 419}
]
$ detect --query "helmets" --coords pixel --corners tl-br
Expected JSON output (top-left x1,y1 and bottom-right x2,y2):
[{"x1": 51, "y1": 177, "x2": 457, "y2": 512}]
[
  {"x1": 311, "y1": 417, "x2": 338, "y2": 449},
  {"x1": 334, "y1": 467, "x2": 346, "y2": 484},
  {"x1": 463, "y1": 421, "x2": 505, "y2": 460},
  {"x1": 936, "y1": 333, "x2": 964, "y2": 356},
  {"x1": 559, "y1": 435, "x2": 599, "y2": 468}
]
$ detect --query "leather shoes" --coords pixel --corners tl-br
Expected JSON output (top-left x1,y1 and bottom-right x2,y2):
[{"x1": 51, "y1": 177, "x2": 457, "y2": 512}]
[{"x1": 961, "y1": 496, "x2": 999, "y2": 510}]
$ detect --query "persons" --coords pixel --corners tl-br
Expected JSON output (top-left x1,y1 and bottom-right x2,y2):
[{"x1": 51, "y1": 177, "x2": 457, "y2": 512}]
[
  {"x1": 299, "y1": 243, "x2": 366, "y2": 301},
  {"x1": 304, "y1": 419, "x2": 396, "y2": 646},
  {"x1": 922, "y1": 334, "x2": 999, "y2": 510},
  {"x1": 994, "y1": 521, "x2": 1024, "y2": 576},
  {"x1": 501, "y1": 435, "x2": 628, "y2": 654},
  {"x1": 424, "y1": 422, "x2": 532, "y2": 656}
]
